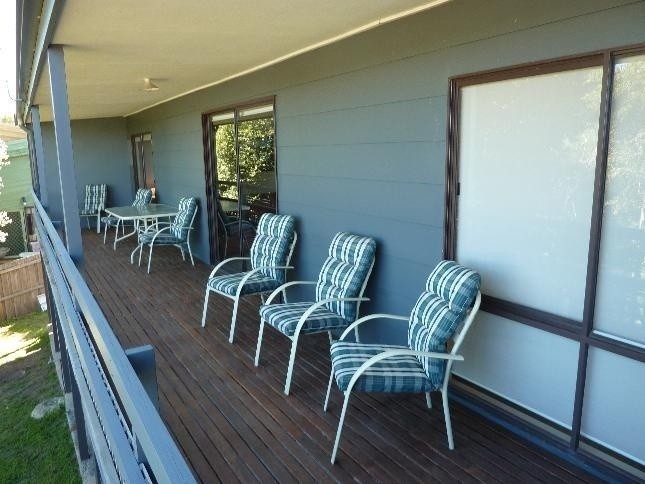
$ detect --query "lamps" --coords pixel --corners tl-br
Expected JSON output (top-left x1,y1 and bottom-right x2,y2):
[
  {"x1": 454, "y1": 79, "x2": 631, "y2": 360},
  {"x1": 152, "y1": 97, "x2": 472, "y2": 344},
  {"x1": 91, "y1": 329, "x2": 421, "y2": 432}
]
[{"x1": 144, "y1": 78, "x2": 159, "y2": 91}]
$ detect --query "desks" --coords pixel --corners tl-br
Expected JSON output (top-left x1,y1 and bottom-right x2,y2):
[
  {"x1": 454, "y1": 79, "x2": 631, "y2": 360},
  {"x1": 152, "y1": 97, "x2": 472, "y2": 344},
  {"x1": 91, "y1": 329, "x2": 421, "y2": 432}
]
[
  {"x1": 104, "y1": 203, "x2": 179, "y2": 264},
  {"x1": 217, "y1": 198, "x2": 250, "y2": 213}
]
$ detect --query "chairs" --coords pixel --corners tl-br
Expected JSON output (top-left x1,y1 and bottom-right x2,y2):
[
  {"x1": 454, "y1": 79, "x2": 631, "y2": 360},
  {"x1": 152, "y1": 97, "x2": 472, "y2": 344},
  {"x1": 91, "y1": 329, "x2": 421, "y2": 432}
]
[
  {"x1": 201, "y1": 212, "x2": 298, "y2": 343},
  {"x1": 101, "y1": 188, "x2": 152, "y2": 245},
  {"x1": 254, "y1": 231, "x2": 376, "y2": 397},
  {"x1": 79, "y1": 184, "x2": 111, "y2": 234},
  {"x1": 217, "y1": 200, "x2": 258, "y2": 263},
  {"x1": 322, "y1": 260, "x2": 481, "y2": 465},
  {"x1": 139, "y1": 196, "x2": 198, "y2": 273}
]
